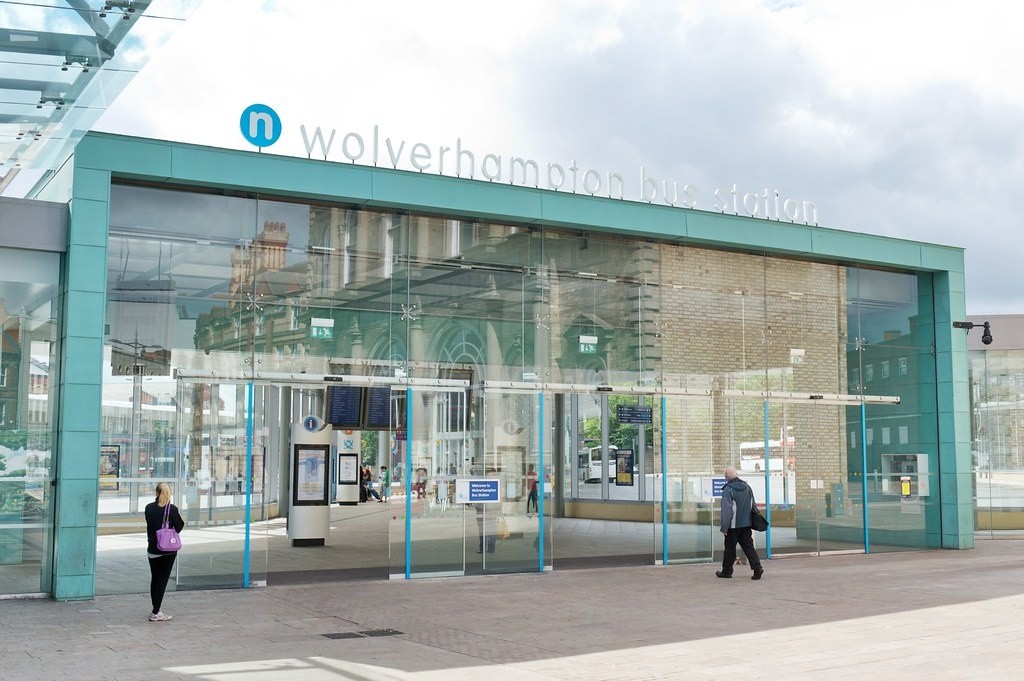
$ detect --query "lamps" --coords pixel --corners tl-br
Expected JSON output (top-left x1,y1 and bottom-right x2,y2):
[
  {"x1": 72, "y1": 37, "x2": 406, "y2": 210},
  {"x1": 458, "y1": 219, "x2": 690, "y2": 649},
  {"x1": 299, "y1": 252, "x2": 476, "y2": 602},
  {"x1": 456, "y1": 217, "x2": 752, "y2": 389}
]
[{"x1": 954, "y1": 321, "x2": 992, "y2": 346}]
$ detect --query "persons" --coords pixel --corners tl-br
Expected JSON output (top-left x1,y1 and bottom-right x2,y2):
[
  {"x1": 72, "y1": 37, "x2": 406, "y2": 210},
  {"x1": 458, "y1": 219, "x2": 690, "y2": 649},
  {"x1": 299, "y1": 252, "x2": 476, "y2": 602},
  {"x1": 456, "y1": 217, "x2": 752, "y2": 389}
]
[
  {"x1": 527, "y1": 464, "x2": 539, "y2": 513},
  {"x1": 416, "y1": 463, "x2": 457, "y2": 503},
  {"x1": 360, "y1": 465, "x2": 390, "y2": 503},
  {"x1": 716, "y1": 467, "x2": 764, "y2": 580},
  {"x1": 475, "y1": 468, "x2": 503, "y2": 554},
  {"x1": 145, "y1": 483, "x2": 185, "y2": 621}
]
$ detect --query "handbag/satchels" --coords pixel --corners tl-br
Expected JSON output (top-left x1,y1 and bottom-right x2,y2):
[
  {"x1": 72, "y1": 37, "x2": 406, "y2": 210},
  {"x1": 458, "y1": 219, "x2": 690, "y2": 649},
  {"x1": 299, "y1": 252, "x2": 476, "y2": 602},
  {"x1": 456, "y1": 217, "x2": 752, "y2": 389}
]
[
  {"x1": 385, "y1": 486, "x2": 390, "y2": 497},
  {"x1": 156, "y1": 501, "x2": 182, "y2": 551},
  {"x1": 496, "y1": 513, "x2": 510, "y2": 542},
  {"x1": 752, "y1": 502, "x2": 769, "y2": 532}
]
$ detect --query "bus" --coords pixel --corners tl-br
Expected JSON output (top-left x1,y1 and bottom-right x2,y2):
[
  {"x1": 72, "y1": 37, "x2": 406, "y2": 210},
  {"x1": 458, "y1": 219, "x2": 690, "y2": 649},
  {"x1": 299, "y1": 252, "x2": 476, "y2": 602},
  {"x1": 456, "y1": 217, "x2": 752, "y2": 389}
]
[{"x1": 578, "y1": 445, "x2": 618, "y2": 483}]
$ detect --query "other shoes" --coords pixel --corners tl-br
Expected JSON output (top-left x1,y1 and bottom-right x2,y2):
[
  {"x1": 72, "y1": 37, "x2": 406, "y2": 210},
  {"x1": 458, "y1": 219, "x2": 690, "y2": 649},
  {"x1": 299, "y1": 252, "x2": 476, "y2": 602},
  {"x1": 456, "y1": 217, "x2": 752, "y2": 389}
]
[
  {"x1": 367, "y1": 498, "x2": 373, "y2": 501},
  {"x1": 377, "y1": 500, "x2": 383, "y2": 502},
  {"x1": 384, "y1": 500, "x2": 388, "y2": 503},
  {"x1": 476, "y1": 549, "x2": 494, "y2": 554}
]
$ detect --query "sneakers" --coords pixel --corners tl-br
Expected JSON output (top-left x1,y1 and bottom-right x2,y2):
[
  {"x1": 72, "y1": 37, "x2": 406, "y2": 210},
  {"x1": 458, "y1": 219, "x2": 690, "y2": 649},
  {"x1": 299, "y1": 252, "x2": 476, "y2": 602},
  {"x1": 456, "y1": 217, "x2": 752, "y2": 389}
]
[
  {"x1": 751, "y1": 568, "x2": 764, "y2": 580},
  {"x1": 716, "y1": 571, "x2": 733, "y2": 578},
  {"x1": 148, "y1": 612, "x2": 173, "y2": 622}
]
